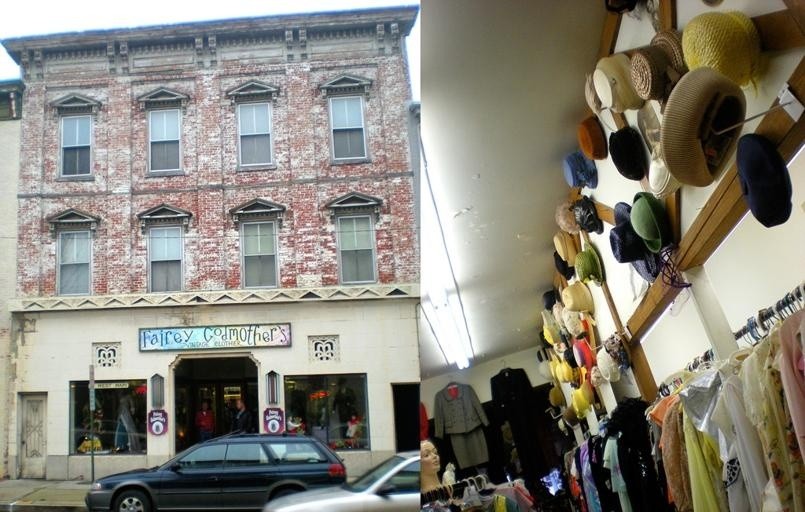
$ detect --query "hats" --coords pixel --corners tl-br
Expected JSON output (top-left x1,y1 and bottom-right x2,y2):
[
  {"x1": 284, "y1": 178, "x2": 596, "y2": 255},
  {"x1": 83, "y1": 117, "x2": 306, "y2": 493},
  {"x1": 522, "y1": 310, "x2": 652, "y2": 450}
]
[
  {"x1": 629, "y1": 30, "x2": 682, "y2": 100},
  {"x1": 537, "y1": 281, "x2": 630, "y2": 436},
  {"x1": 609, "y1": 202, "x2": 662, "y2": 283},
  {"x1": 562, "y1": 151, "x2": 598, "y2": 189},
  {"x1": 554, "y1": 200, "x2": 578, "y2": 234},
  {"x1": 574, "y1": 239, "x2": 604, "y2": 286},
  {"x1": 735, "y1": 132, "x2": 792, "y2": 229},
  {"x1": 573, "y1": 195, "x2": 604, "y2": 234},
  {"x1": 680, "y1": 10, "x2": 763, "y2": 88},
  {"x1": 576, "y1": 114, "x2": 608, "y2": 160},
  {"x1": 630, "y1": 191, "x2": 673, "y2": 253},
  {"x1": 592, "y1": 53, "x2": 645, "y2": 112},
  {"x1": 639, "y1": 145, "x2": 681, "y2": 199},
  {"x1": 584, "y1": 74, "x2": 601, "y2": 115},
  {"x1": 658, "y1": 65, "x2": 746, "y2": 187},
  {"x1": 554, "y1": 251, "x2": 575, "y2": 280},
  {"x1": 553, "y1": 230, "x2": 583, "y2": 268},
  {"x1": 609, "y1": 126, "x2": 646, "y2": 181}
]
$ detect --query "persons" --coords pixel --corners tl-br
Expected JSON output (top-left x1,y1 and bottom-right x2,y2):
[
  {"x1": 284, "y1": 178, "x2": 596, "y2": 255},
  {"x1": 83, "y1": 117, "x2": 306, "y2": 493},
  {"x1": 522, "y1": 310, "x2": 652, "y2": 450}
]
[
  {"x1": 347, "y1": 415, "x2": 362, "y2": 447},
  {"x1": 422, "y1": 440, "x2": 440, "y2": 492},
  {"x1": 333, "y1": 379, "x2": 359, "y2": 438},
  {"x1": 115, "y1": 388, "x2": 137, "y2": 451},
  {"x1": 196, "y1": 400, "x2": 215, "y2": 440},
  {"x1": 639, "y1": 117, "x2": 663, "y2": 158},
  {"x1": 233, "y1": 399, "x2": 254, "y2": 431}
]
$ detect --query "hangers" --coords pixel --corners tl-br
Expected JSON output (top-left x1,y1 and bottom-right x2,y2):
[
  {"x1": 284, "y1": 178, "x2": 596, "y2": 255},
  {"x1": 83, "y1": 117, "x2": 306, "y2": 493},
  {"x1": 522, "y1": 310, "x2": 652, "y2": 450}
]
[
  {"x1": 421, "y1": 470, "x2": 490, "y2": 512},
  {"x1": 447, "y1": 374, "x2": 458, "y2": 390},
  {"x1": 657, "y1": 280, "x2": 805, "y2": 398},
  {"x1": 501, "y1": 359, "x2": 512, "y2": 373}
]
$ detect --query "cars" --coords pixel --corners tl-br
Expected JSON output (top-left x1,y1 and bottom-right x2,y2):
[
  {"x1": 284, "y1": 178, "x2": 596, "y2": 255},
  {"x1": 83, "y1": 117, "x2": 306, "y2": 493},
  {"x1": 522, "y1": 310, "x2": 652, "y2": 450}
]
[
  {"x1": 264, "y1": 451, "x2": 421, "y2": 511},
  {"x1": 84, "y1": 436, "x2": 347, "y2": 511}
]
